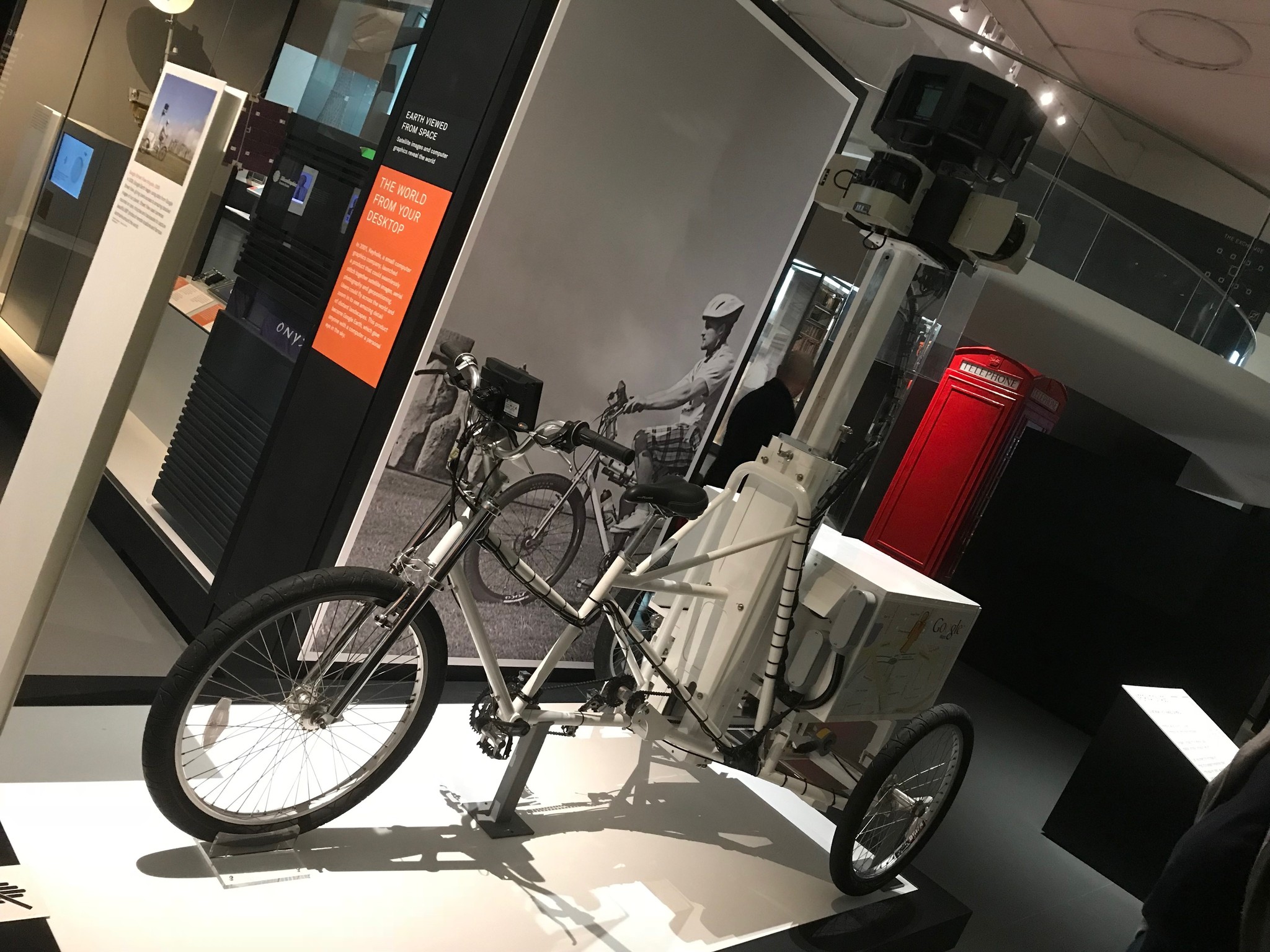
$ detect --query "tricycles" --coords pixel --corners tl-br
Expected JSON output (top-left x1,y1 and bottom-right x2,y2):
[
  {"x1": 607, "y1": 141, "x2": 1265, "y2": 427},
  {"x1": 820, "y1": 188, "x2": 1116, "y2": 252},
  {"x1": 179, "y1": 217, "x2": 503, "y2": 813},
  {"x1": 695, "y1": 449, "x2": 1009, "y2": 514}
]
[{"x1": 135, "y1": 336, "x2": 979, "y2": 899}]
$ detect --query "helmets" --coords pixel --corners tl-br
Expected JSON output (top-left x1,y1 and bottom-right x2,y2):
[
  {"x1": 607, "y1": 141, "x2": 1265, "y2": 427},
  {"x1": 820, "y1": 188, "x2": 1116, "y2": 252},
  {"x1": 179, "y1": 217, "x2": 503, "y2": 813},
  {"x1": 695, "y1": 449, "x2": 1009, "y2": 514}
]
[{"x1": 701, "y1": 293, "x2": 745, "y2": 321}]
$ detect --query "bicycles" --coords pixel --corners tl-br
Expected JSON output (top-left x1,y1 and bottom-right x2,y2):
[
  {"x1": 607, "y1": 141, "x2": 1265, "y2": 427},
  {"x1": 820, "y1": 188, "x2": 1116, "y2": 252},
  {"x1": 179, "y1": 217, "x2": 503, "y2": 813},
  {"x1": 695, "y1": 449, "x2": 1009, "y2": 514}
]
[
  {"x1": 139, "y1": 137, "x2": 168, "y2": 162},
  {"x1": 462, "y1": 380, "x2": 686, "y2": 606}
]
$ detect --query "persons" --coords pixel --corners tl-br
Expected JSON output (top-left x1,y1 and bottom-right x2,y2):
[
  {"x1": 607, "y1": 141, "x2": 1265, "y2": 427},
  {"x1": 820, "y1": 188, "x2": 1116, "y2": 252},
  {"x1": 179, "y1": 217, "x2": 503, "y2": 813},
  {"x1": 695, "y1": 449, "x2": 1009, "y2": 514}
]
[
  {"x1": 292, "y1": 174, "x2": 308, "y2": 202},
  {"x1": 158, "y1": 118, "x2": 170, "y2": 148},
  {"x1": 345, "y1": 198, "x2": 359, "y2": 221},
  {"x1": 577, "y1": 292, "x2": 746, "y2": 589},
  {"x1": 703, "y1": 349, "x2": 814, "y2": 494}
]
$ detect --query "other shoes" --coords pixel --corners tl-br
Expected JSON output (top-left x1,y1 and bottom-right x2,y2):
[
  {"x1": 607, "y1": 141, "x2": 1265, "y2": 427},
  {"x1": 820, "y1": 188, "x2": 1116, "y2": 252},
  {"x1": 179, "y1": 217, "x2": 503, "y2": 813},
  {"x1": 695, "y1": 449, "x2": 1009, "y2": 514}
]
[
  {"x1": 610, "y1": 501, "x2": 651, "y2": 533},
  {"x1": 574, "y1": 576, "x2": 598, "y2": 589}
]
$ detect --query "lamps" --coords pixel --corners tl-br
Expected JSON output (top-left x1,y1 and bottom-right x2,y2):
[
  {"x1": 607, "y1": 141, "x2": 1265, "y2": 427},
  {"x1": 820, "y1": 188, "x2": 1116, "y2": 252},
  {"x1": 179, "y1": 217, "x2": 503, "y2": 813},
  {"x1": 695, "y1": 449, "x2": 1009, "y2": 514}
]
[
  {"x1": 961, "y1": 0, "x2": 1006, "y2": 52},
  {"x1": 147, "y1": 0, "x2": 194, "y2": 70}
]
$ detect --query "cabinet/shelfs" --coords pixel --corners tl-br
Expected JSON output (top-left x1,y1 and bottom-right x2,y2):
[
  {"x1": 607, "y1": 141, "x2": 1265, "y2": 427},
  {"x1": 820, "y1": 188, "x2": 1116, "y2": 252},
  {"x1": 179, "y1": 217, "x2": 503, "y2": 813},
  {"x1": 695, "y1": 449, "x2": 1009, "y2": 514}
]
[{"x1": 743, "y1": 270, "x2": 845, "y2": 404}]
